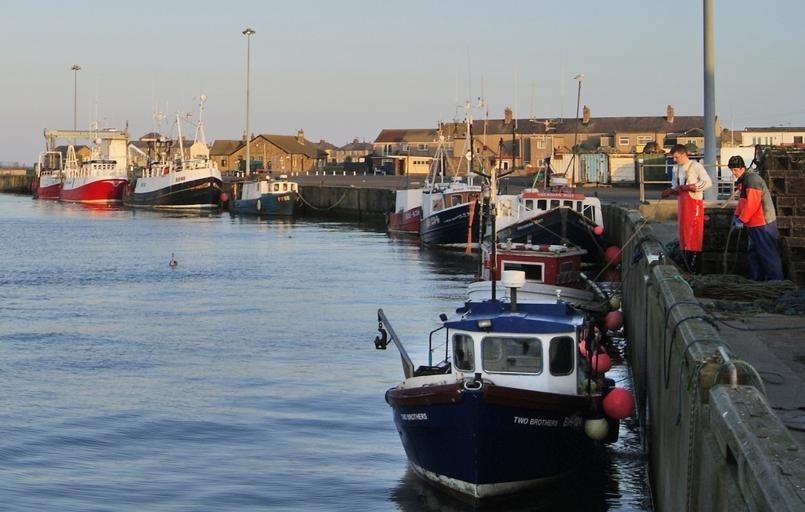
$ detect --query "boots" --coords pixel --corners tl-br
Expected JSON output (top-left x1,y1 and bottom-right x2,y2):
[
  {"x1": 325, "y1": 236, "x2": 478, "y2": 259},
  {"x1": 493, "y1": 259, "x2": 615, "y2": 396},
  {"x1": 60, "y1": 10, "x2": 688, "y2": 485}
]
[{"x1": 678, "y1": 254, "x2": 697, "y2": 272}]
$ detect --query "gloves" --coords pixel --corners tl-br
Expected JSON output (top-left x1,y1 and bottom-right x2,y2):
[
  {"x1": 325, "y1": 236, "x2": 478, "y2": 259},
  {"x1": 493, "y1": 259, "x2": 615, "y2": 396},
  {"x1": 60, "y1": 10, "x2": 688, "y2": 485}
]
[
  {"x1": 661, "y1": 188, "x2": 673, "y2": 198},
  {"x1": 676, "y1": 184, "x2": 696, "y2": 194},
  {"x1": 732, "y1": 215, "x2": 744, "y2": 230}
]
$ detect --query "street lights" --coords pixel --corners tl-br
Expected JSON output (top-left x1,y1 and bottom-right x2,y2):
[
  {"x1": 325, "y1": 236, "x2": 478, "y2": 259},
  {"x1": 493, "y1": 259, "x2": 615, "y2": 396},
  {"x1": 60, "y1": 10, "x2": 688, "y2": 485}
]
[
  {"x1": 241, "y1": 27, "x2": 256, "y2": 175},
  {"x1": 572, "y1": 73, "x2": 585, "y2": 185}
]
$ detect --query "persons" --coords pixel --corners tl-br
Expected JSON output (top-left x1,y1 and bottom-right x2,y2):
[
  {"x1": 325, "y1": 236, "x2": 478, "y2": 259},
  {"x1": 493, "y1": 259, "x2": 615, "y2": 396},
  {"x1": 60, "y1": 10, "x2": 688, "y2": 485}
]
[
  {"x1": 661, "y1": 144, "x2": 713, "y2": 274},
  {"x1": 728, "y1": 155, "x2": 783, "y2": 282}
]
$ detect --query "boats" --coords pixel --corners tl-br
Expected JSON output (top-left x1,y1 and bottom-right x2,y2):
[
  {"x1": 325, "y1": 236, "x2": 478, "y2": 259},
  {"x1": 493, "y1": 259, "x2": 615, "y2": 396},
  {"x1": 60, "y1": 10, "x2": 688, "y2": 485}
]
[
  {"x1": 228, "y1": 175, "x2": 299, "y2": 215},
  {"x1": 71, "y1": 64, "x2": 81, "y2": 130},
  {"x1": 35, "y1": 94, "x2": 225, "y2": 210}
]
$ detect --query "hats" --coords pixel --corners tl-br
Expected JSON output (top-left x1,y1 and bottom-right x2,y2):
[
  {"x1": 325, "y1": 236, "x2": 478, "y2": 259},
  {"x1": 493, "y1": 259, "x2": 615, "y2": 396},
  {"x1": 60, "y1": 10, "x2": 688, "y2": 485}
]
[{"x1": 728, "y1": 156, "x2": 744, "y2": 168}]
